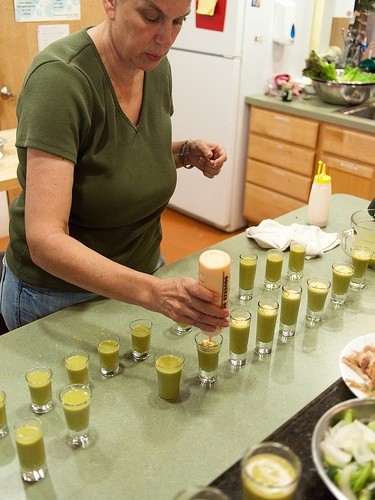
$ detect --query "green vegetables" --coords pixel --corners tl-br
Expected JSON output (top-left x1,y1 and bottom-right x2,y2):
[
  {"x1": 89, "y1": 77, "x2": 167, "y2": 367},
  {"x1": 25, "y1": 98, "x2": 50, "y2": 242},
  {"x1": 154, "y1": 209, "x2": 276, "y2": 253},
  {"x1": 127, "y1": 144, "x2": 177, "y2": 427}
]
[{"x1": 320, "y1": 410, "x2": 375, "y2": 500}]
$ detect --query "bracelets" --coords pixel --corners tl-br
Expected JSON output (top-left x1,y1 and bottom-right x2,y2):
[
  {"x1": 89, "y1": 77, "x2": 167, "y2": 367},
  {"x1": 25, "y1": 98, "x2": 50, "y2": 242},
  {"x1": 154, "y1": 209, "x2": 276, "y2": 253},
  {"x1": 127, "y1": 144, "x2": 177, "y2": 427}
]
[{"x1": 180, "y1": 138, "x2": 194, "y2": 169}]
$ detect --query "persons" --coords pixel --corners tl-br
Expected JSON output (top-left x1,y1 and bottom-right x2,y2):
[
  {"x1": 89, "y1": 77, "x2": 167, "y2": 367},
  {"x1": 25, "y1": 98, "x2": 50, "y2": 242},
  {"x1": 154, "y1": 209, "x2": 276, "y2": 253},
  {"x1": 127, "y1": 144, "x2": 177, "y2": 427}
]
[{"x1": 0, "y1": 0, "x2": 230, "y2": 332}]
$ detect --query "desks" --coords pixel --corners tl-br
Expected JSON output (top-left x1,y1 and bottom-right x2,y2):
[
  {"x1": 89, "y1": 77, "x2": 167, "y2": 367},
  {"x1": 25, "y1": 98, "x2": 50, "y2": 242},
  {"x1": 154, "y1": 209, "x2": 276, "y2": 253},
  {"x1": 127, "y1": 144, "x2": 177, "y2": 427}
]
[
  {"x1": 0, "y1": 192, "x2": 375, "y2": 500},
  {"x1": 0, "y1": 128, "x2": 23, "y2": 204}
]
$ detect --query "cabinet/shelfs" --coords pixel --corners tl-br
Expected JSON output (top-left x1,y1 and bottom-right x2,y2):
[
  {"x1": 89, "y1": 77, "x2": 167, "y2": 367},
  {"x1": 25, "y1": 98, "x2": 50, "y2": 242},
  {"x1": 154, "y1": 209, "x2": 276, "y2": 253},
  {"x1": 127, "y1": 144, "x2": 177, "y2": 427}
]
[{"x1": 243, "y1": 104, "x2": 375, "y2": 226}]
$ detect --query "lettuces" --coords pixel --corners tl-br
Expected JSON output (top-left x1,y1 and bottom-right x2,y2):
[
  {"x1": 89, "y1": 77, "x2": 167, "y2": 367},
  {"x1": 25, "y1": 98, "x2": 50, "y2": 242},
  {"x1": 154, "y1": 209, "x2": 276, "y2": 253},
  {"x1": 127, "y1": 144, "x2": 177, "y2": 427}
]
[{"x1": 302, "y1": 49, "x2": 375, "y2": 83}]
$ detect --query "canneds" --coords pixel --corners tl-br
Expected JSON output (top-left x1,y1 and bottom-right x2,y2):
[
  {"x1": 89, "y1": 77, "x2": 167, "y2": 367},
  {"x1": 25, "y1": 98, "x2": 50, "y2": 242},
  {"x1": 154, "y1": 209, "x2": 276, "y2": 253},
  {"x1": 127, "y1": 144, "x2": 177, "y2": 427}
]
[{"x1": 282, "y1": 85, "x2": 293, "y2": 102}]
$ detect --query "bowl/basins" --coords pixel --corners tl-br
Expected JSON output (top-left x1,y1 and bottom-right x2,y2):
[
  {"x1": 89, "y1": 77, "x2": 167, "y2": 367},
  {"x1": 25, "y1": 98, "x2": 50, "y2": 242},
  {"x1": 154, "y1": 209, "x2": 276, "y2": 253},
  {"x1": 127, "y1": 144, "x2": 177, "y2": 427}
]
[
  {"x1": 313, "y1": 69, "x2": 375, "y2": 106},
  {"x1": 311, "y1": 397, "x2": 375, "y2": 500}
]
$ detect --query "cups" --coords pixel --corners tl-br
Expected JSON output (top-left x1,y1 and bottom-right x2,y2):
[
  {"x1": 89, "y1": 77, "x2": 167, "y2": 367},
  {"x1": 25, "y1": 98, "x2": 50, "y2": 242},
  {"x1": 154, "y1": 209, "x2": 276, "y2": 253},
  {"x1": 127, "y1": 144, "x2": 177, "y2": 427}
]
[
  {"x1": 330, "y1": 262, "x2": 355, "y2": 304},
  {"x1": 229, "y1": 308, "x2": 251, "y2": 366},
  {"x1": 341, "y1": 209, "x2": 375, "y2": 269},
  {"x1": 154, "y1": 350, "x2": 185, "y2": 401},
  {"x1": 59, "y1": 383, "x2": 92, "y2": 449},
  {"x1": 255, "y1": 299, "x2": 279, "y2": 354},
  {"x1": 195, "y1": 332, "x2": 223, "y2": 384},
  {"x1": 279, "y1": 283, "x2": 303, "y2": 337},
  {"x1": 63, "y1": 349, "x2": 91, "y2": 387},
  {"x1": 96, "y1": 319, "x2": 153, "y2": 377},
  {"x1": 0, "y1": 391, "x2": 9, "y2": 438},
  {"x1": 174, "y1": 486, "x2": 230, "y2": 500},
  {"x1": 241, "y1": 442, "x2": 302, "y2": 500},
  {"x1": 239, "y1": 240, "x2": 307, "y2": 301},
  {"x1": 306, "y1": 277, "x2": 331, "y2": 322},
  {"x1": 174, "y1": 322, "x2": 192, "y2": 336},
  {"x1": 350, "y1": 247, "x2": 373, "y2": 291},
  {"x1": 25, "y1": 365, "x2": 54, "y2": 413},
  {"x1": 14, "y1": 417, "x2": 48, "y2": 482}
]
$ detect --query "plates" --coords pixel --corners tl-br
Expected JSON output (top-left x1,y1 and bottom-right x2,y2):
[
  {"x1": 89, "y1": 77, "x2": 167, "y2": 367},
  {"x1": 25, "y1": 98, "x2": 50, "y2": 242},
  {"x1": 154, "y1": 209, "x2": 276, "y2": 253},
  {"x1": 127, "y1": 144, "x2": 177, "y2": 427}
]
[{"x1": 339, "y1": 332, "x2": 375, "y2": 398}]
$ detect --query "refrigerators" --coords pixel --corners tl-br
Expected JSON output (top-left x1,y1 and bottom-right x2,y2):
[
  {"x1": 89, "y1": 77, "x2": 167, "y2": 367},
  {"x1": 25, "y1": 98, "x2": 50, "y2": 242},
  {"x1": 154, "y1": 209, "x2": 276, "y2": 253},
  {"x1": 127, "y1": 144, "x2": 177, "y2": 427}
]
[{"x1": 166, "y1": 0, "x2": 316, "y2": 232}]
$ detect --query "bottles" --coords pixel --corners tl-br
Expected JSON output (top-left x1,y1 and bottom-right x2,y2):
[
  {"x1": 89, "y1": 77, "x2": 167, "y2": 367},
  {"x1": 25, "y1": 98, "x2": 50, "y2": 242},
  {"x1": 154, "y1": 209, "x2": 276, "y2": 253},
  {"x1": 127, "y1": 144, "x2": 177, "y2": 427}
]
[
  {"x1": 307, "y1": 161, "x2": 331, "y2": 227},
  {"x1": 282, "y1": 83, "x2": 293, "y2": 102},
  {"x1": 198, "y1": 249, "x2": 231, "y2": 342}
]
